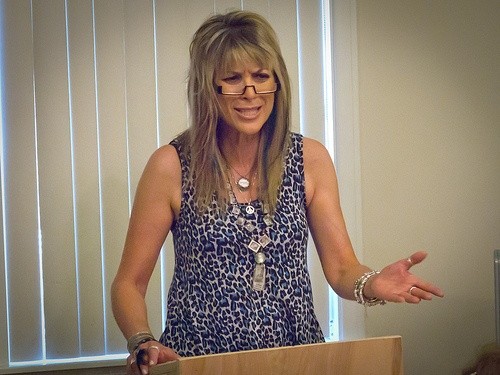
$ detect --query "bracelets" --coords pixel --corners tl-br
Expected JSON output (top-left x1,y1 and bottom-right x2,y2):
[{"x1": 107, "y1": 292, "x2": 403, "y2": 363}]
[
  {"x1": 353, "y1": 270, "x2": 387, "y2": 306},
  {"x1": 133, "y1": 337, "x2": 157, "y2": 351},
  {"x1": 127, "y1": 331, "x2": 154, "y2": 354}
]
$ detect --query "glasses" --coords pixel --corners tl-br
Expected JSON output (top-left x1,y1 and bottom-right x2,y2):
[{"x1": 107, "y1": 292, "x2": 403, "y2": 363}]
[{"x1": 212, "y1": 82, "x2": 281, "y2": 95}]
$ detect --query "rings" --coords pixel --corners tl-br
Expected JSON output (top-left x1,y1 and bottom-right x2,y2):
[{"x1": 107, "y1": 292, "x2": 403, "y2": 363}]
[
  {"x1": 148, "y1": 346, "x2": 160, "y2": 352},
  {"x1": 408, "y1": 286, "x2": 417, "y2": 295},
  {"x1": 408, "y1": 258, "x2": 414, "y2": 265}
]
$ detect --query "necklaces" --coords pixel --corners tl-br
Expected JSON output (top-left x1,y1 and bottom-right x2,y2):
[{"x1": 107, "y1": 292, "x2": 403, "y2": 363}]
[{"x1": 223, "y1": 158, "x2": 275, "y2": 292}]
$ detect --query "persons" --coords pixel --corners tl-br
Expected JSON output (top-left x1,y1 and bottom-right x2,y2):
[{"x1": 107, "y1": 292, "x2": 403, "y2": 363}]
[{"x1": 110, "y1": 10, "x2": 445, "y2": 375}]
